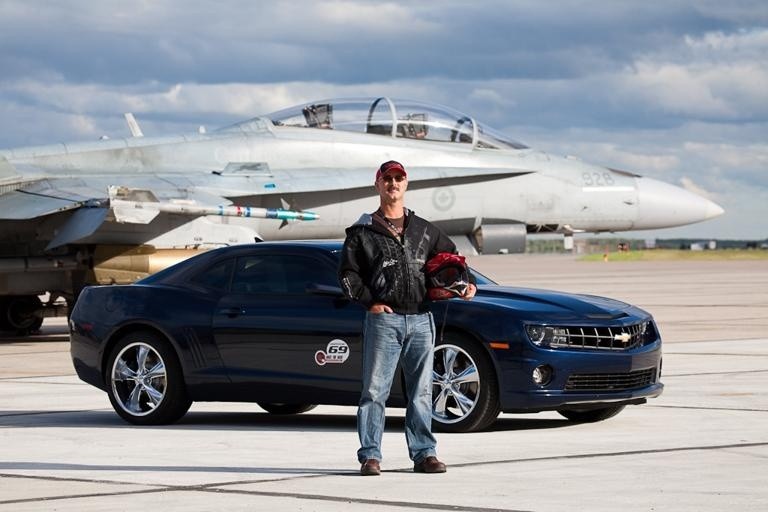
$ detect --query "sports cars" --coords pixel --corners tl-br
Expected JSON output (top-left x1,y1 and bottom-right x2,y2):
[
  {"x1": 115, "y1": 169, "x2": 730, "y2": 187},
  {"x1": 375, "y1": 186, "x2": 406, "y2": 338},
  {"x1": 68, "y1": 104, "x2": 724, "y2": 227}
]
[{"x1": 68, "y1": 240, "x2": 663, "y2": 433}]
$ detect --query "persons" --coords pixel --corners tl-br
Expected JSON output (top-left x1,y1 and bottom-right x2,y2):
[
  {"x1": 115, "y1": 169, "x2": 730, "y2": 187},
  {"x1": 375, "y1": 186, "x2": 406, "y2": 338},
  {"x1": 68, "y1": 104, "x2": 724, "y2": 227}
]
[{"x1": 338, "y1": 160, "x2": 476, "y2": 474}]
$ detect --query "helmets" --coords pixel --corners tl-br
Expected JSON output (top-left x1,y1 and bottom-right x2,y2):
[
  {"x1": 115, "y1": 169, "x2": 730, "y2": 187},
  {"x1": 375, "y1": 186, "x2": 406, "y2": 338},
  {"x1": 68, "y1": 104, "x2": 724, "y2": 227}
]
[{"x1": 425, "y1": 252, "x2": 470, "y2": 299}]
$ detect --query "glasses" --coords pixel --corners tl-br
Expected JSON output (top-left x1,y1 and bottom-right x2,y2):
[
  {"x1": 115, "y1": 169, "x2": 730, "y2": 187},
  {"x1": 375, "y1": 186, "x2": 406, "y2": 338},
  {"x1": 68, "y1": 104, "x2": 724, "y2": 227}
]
[{"x1": 378, "y1": 176, "x2": 406, "y2": 182}]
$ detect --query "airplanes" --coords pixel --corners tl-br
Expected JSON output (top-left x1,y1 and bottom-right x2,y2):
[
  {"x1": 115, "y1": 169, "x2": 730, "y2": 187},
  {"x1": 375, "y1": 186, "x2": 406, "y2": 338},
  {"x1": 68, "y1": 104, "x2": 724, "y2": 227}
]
[{"x1": 1, "y1": 96, "x2": 725, "y2": 340}]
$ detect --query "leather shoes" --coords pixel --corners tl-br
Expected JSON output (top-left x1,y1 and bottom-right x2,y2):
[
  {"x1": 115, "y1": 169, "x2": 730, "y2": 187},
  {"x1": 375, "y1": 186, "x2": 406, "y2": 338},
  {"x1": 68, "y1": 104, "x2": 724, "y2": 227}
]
[
  {"x1": 361, "y1": 459, "x2": 380, "y2": 475},
  {"x1": 414, "y1": 458, "x2": 446, "y2": 473}
]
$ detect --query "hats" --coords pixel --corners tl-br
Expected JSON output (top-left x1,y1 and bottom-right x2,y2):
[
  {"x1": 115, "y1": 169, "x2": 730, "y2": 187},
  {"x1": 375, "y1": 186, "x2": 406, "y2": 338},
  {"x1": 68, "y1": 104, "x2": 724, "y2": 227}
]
[{"x1": 376, "y1": 161, "x2": 406, "y2": 179}]
